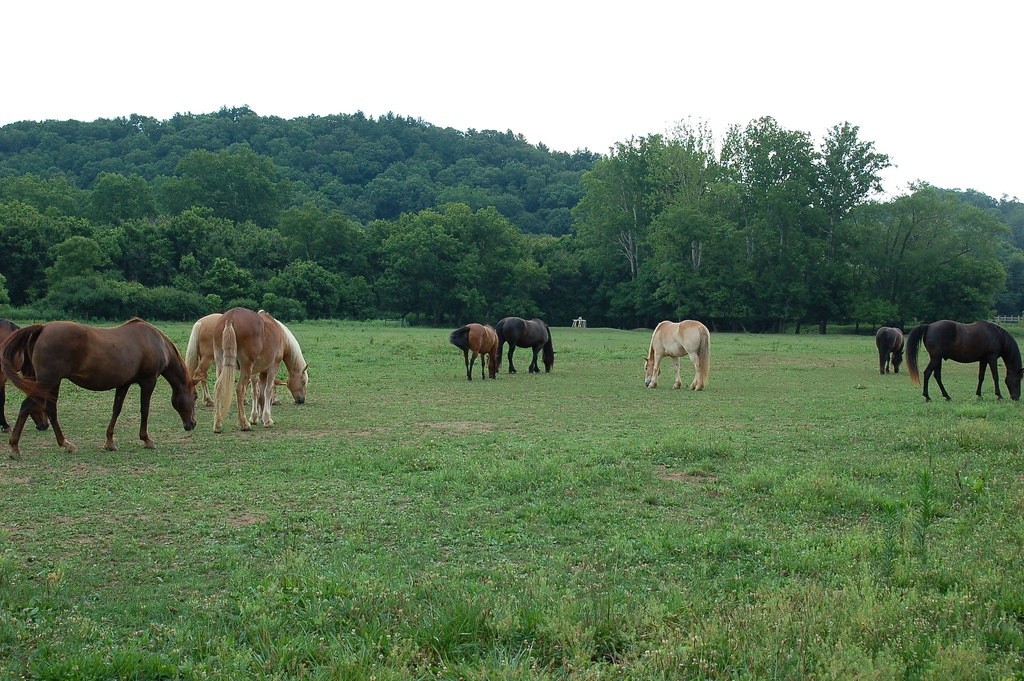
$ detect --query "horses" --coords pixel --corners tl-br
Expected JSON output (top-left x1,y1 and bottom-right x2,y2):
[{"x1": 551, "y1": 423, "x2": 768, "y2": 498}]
[
  {"x1": 876, "y1": 327, "x2": 905, "y2": 374},
  {"x1": 450, "y1": 316, "x2": 554, "y2": 381},
  {"x1": 905, "y1": 319, "x2": 1023, "y2": 402},
  {"x1": 186, "y1": 307, "x2": 309, "y2": 433},
  {"x1": 642, "y1": 320, "x2": 710, "y2": 391},
  {"x1": 0, "y1": 315, "x2": 198, "y2": 459}
]
